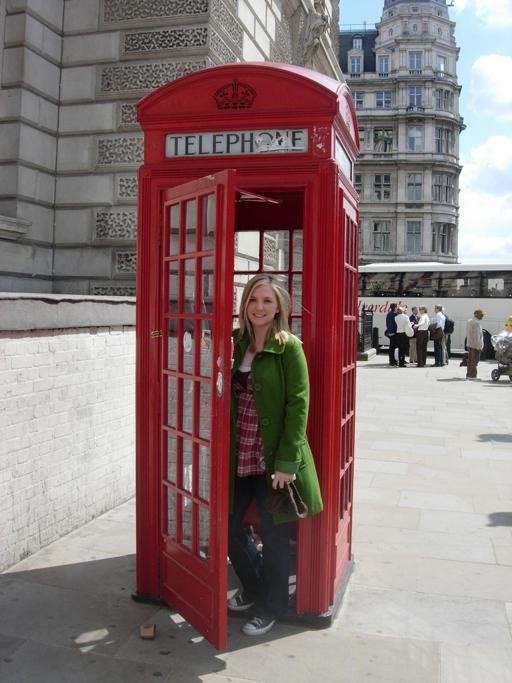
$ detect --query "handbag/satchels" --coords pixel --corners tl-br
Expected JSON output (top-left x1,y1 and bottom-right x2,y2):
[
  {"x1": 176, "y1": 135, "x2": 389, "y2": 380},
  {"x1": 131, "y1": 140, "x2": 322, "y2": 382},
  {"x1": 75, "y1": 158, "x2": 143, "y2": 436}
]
[{"x1": 385, "y1": 327, "x2": 397, "y2": 337}]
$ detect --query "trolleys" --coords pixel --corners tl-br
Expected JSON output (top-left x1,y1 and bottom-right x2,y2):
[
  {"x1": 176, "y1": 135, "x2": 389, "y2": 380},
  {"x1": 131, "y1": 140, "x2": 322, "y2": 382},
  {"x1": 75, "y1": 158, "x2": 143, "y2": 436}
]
[{"x1": 489, "y1": 328, "x2": 512, "y2": 383}]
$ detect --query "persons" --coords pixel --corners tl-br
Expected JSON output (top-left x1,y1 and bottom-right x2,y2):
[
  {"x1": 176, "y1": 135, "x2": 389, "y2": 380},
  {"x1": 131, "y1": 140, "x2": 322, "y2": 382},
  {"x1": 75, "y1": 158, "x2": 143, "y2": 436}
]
[
  {"x1": 464, "y1": 307, "x2": 486, "y2": 380},
  {"x1": 225, "y1": 270, "x2": 324, "y2": 638},
  {"x1": 384, "y1": 301, "x2": 454, "y2": 367}
]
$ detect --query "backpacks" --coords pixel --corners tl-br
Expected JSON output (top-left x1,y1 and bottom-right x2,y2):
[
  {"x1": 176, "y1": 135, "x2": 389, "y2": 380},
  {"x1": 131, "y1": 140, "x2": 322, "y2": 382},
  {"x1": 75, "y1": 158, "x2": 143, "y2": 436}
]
[{"x1": 442, "y1": 313, "x2": 454, "y2": 335}]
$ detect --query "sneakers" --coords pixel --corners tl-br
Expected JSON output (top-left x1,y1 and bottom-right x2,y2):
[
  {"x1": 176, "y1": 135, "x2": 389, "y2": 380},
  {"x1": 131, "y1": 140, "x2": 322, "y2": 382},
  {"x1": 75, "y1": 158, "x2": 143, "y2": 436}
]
[
  {"x1": 227, "y1": 590, "x2": 258, "y2": 612},
  {"x1": 241, "y1": 612, "x2": 277, "y2": 638},
  {"x1": 389, "y1": 359, "x2": 482, "y2": 380}
]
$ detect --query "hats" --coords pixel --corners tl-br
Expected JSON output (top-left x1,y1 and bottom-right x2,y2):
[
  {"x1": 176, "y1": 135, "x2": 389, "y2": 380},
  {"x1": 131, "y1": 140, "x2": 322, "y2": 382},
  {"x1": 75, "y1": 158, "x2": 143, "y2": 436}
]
[{"x1": 265, "y1": 477, "x2": 309, "y2": 520}]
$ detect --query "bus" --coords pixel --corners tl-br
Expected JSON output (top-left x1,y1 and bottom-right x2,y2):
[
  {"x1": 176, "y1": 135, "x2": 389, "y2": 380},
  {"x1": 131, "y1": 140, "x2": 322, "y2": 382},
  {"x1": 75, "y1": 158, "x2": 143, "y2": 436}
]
[{"x1": 354, "y1": 260, "x2": 512, "y2": 353}]
